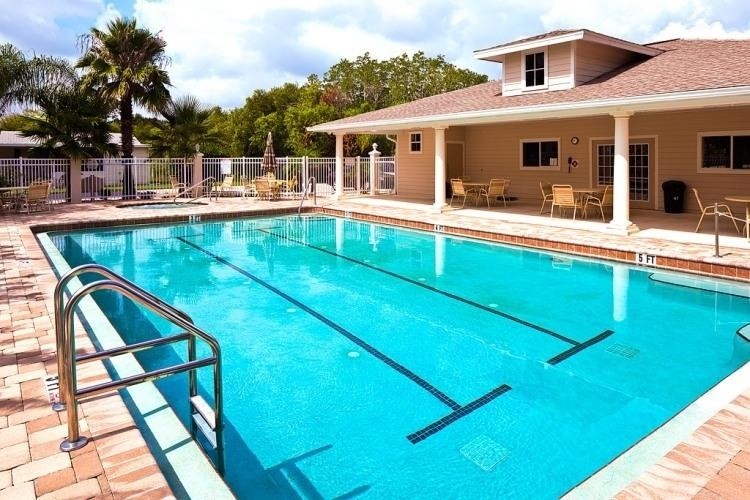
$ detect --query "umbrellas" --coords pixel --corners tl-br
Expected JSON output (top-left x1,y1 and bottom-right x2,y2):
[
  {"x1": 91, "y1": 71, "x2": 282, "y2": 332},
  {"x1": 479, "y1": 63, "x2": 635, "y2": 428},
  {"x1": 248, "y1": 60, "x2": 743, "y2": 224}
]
[{"x1": 261, "y1": 132, "x2": 278, "y2": 182}]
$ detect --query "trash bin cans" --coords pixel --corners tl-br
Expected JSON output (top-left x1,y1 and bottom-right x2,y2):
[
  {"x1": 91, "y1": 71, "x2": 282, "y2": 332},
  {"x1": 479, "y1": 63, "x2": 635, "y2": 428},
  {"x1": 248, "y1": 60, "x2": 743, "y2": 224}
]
[{"x1": 662, "y1": 180, "x2": 685, "y2": 213}]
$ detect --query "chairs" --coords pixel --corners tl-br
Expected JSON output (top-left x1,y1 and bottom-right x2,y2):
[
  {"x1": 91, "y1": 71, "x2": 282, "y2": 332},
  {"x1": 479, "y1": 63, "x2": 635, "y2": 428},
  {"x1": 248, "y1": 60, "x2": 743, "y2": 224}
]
[
  {"x1": 0, "y1": 177, "x2": 56, "y2": 218},
  {"x1": 537, "y1": 179, "x2": 611, "y2": 224},
  {"x1": 239, "y1": 172, "x2": 298, "y2": 202},
  {"x1": 446, "y1": 175, "x2": 512, "y2": 211},
  {"x1": 168, "y1": 174, "x2": 189, "y2": 198},
  {"x1": 209, "y1": 175, "x2": 234, "y2": 198}
]
